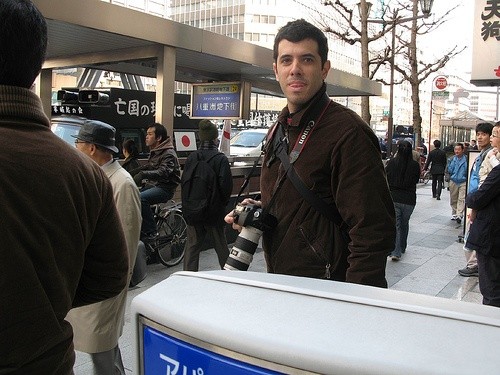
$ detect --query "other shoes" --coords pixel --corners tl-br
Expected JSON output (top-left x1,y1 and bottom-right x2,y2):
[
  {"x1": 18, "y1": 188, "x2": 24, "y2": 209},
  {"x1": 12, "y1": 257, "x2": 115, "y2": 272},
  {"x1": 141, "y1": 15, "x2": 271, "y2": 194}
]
[
  {"x1": 145, "y1": 231, "x2": 160, "y2": 240},
  {"x1": 456, "y1": 216, "x2": 462, "y2": 219},
  {"x1": 458, "y1": 267, "x2": 479, "y2": 277},
  {"x1": 437, "y1": 197, "x2": 440, "y2": 200},
  {"x1": 451, "y1": 215, "x2": 456, "y2": 220},
  {"x1": 392, "y1": 256, "x2": 399, "y2": 261}
]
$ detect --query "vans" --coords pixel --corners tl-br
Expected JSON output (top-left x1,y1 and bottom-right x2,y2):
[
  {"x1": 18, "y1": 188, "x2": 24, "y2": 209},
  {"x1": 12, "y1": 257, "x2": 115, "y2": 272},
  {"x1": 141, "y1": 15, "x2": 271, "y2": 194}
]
[{"x1": 230, "y1": 128, "x2": 269, "y2": 167}]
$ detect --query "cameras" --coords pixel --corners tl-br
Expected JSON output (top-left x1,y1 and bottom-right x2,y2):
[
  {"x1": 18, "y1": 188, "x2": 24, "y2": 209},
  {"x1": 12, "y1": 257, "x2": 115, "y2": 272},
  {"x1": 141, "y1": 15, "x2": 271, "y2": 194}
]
[{"x1": 222, "y1": 202, "x2": 278, "y2": 270}]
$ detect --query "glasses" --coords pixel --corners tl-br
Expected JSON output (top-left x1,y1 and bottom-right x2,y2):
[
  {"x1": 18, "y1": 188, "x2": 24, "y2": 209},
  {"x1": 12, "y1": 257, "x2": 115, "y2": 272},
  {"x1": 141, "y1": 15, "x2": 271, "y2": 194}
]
[{"x1": 74, "y1": 140, "x2": 87, "y2": 146}]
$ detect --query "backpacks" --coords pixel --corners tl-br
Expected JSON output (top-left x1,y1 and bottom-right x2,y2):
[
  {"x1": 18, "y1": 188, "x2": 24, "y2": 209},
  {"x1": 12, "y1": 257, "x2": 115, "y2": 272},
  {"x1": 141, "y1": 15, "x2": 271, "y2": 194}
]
[{"x1": 182, "y1": 152, "x2": 224, "y2": 226}]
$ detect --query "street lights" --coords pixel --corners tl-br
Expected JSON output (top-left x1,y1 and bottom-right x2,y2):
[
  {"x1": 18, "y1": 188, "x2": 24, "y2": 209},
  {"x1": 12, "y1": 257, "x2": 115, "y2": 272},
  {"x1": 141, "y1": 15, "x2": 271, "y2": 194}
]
[{"x1": 357, "y1": 0, "x2": 436, "y2": 159}]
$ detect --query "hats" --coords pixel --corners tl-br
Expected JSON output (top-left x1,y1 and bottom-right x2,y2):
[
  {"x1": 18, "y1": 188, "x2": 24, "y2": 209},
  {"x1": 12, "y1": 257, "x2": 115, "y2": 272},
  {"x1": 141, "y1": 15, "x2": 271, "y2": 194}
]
[
  {"x1": 198, "y1": 119, "x2": 218, "y2": 142},
  {"x1": 70, "y1": 120, "x2": 119, "y2": 153}
]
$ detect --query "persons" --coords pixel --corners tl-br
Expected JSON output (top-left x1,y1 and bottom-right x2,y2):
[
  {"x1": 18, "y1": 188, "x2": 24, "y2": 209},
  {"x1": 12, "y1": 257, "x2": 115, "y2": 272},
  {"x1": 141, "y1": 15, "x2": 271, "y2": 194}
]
[
  {"x1": 424, "y1": 140, "x2": 447, "y2": 199},
  {"x1": 445, "y1": 121, "x2": 500, "y2": 308},
  {"x1": 385, "y1": 140, "x2": 421, "y2": 260},
  {"x1": 65, "y1": 120, "x2": 144, "y2": 375},
  {"x1": 224, "y1": 18, "x2": 398, "y2": 290},
  {"x1": 180, "y1": 119, "x2": 233, "y2": 272},
  {"x1": 120, "y1": 139, "x2": 138, "y2": 166},
  {"x1": 128, "y1": 123, "x2": 182, "y2": 241},
  {"x1": 0, "y1": 0, "x2": 130, "y2": 375}
]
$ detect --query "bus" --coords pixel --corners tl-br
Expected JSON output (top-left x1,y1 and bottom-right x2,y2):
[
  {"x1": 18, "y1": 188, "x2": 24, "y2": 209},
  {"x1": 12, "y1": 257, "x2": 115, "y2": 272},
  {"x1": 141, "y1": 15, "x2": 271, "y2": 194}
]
[{"x1": 50, "y1": 85, "x2": 203, "y2": 168}]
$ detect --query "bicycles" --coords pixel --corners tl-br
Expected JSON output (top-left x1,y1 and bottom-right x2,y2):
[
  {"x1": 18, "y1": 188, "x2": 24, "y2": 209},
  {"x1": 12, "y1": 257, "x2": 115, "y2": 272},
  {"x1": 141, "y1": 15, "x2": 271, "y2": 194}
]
[{"x1": 141, "y1": 201, "x2": 190, "y2": 267}]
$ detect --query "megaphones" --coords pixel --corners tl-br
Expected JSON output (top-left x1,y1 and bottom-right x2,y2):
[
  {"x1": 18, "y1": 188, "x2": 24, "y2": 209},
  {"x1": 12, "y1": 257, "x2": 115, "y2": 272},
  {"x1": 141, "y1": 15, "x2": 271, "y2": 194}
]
[{"x1": 55, "y1": 89, "x2": 110, "y2": 104}]
